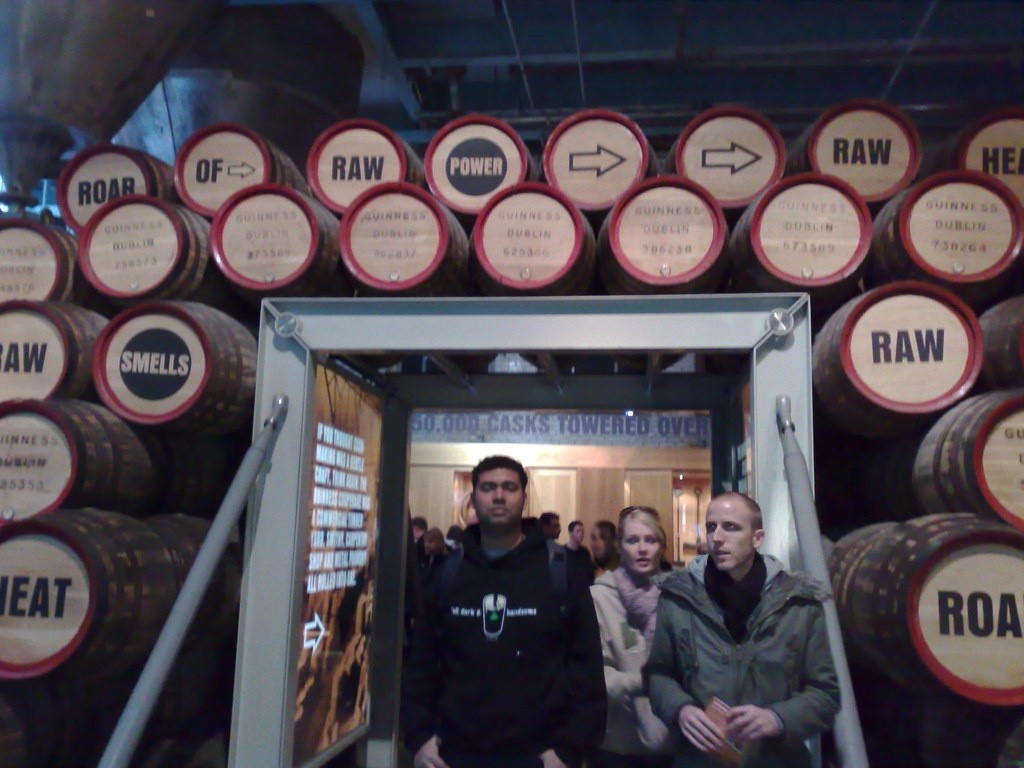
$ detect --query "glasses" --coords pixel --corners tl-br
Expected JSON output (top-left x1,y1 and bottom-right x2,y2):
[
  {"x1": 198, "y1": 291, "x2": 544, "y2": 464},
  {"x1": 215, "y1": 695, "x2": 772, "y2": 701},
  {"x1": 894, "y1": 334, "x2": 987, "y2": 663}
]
[{"x1": 619, "y1": 505, "x2": 656, "y2": 520}]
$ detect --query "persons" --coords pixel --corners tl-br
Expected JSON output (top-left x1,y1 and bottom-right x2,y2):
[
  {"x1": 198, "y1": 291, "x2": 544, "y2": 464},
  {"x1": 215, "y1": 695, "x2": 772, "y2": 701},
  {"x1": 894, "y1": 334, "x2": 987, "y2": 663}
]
[
  {"x1": 402, "y1": 512, "x2": 620, "y2": 665},
  {"x1": 395, "y1": 457, "x2": 613, "y2": 768},
  {"x1": 590, "y1": 506, "x2": 688, "y2": 768},
  {"x1": 648, "y1": 492, "x2": 841, "y2": 768}
]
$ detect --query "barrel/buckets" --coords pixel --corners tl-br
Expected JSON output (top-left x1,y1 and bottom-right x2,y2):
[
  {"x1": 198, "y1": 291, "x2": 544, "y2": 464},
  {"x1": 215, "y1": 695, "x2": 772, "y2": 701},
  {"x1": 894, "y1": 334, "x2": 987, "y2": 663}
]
[{"x1": 1, "y1": 99, "x2": 1024, "y2": 768}]
